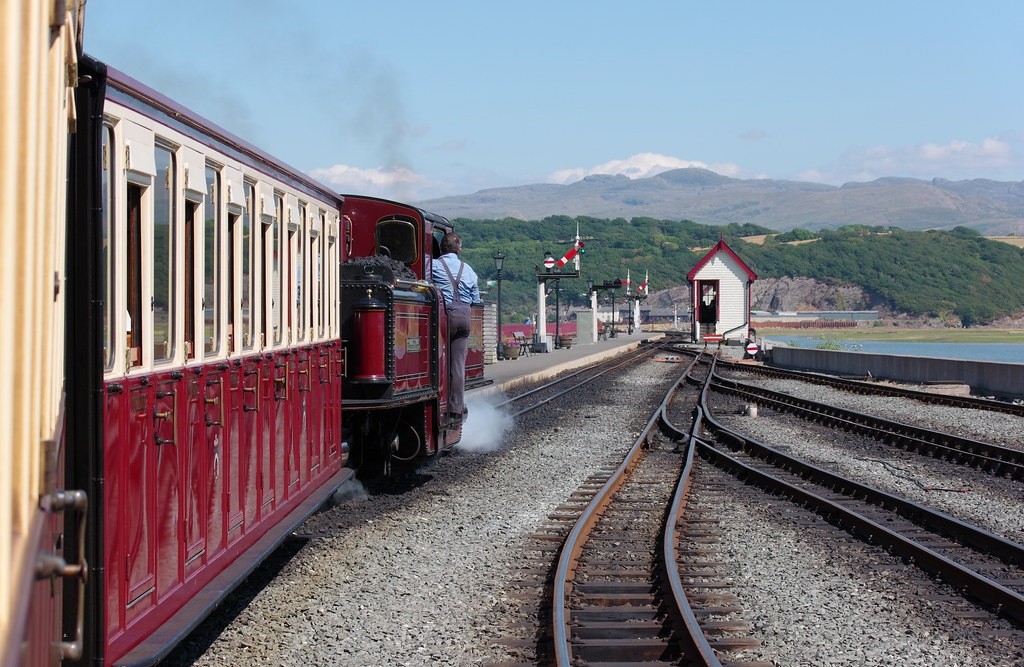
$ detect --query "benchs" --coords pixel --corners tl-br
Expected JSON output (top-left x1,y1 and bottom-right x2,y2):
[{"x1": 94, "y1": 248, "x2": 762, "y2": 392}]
[
  {"x1": 512, "y1": 332, "x2": 537, "y2": 356},
  {"x1": 598, "y1": 325, "x2": 610, "y2": 341}
]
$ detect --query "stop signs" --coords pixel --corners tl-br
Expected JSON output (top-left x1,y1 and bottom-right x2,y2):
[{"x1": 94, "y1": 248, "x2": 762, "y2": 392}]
[
  {"x1": 747, "y1": 343, "x2": 758, "y2": 354},
  {"x1": 543, "y1": 257, "x2": 555, "y2": 268}
]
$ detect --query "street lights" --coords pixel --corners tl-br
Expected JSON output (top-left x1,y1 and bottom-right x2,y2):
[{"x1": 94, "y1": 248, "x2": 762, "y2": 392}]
[
  {"x1": 554, "y1": 267, "x2": 561, "y2": 349},
  {"x1": 493, "y1": 249, "x2": 506, "y2": 362},
  {"x1": 587, "y1": 278, "x2": 594, "y2": 308},
  {"x1": 611, "y1": 289, "x2": 615, "y2": 338}
]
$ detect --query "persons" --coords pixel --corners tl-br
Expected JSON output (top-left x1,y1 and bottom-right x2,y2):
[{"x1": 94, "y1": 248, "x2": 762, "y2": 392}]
[{"x1": 431, "y1": 232, "x2": 481, "y2": 419}]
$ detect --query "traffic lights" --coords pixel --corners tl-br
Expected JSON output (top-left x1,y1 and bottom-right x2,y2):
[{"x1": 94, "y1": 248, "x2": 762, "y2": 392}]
[{"x1": 581, "y1": 249, "x2": 585, "y2": 254}]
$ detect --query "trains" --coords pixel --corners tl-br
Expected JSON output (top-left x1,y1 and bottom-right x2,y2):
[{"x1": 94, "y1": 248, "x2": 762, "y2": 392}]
[{"x1": 0, "y1": 0, "x2": 468, "y2": 667}]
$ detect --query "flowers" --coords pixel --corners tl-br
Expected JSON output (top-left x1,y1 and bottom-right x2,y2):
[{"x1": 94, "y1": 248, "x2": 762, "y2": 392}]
[
  {"x1": 562, "y1": 321, "x2": 572, "y2": 336},
  {"x1": 501, "y1": 336, "x2": 521, "y2": 347}
]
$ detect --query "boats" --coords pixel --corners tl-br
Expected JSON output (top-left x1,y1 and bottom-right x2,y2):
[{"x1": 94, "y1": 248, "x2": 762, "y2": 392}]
[{"x1": 648, "y1": 281, "x2": 651, "y2": 286}]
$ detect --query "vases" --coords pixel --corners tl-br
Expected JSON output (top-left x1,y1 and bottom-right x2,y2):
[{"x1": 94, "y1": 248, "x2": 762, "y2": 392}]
[
  {"x1": 557, "y1": 335, "x2": 572, "y2": 347},
  {"x1": 503, "y1": 346, "x2": 520, "y2": 358}
]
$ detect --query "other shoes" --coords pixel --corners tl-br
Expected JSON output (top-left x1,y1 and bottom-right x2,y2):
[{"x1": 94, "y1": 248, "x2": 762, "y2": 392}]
[{"x1": 450, "y1": 403, "x2": 467, "y2": 418}]
